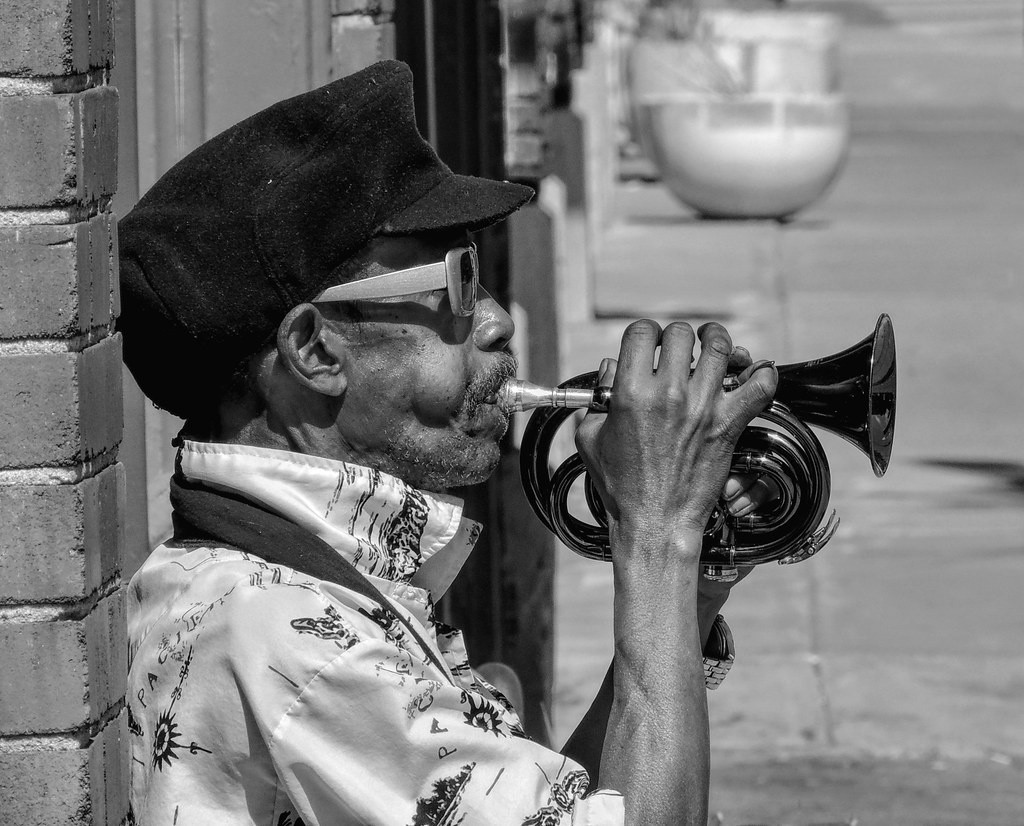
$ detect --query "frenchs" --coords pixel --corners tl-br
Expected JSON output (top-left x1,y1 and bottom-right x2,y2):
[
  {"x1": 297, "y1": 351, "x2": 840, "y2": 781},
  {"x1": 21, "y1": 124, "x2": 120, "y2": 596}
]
[{"x1": 493, "y1": 309, "x2": 898, "y2": 585}]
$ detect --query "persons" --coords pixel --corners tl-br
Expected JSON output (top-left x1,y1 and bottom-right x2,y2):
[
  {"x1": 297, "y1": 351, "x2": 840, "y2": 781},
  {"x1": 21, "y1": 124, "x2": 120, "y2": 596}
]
[{"x1": 117, "y1": 61, "x2": 779, "y2": 826}]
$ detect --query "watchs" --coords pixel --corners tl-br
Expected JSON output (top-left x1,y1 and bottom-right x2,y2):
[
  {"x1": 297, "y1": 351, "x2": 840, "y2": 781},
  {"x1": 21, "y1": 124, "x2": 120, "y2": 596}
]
[{"x1": 702, "y1": 613, "x2": 735, "y2": 690}]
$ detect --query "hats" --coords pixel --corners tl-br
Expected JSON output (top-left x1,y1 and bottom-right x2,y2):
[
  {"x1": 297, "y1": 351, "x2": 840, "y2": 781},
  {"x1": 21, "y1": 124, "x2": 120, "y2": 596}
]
[{"x1": 118, "y1": 60, "x2": 536, "y2": 411}]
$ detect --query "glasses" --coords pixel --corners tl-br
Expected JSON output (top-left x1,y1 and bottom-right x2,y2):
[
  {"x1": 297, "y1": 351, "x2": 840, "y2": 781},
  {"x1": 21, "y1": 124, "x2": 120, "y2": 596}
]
[{"x1": 306, "y1": 242, "x2": 481, "y2": 317}]
